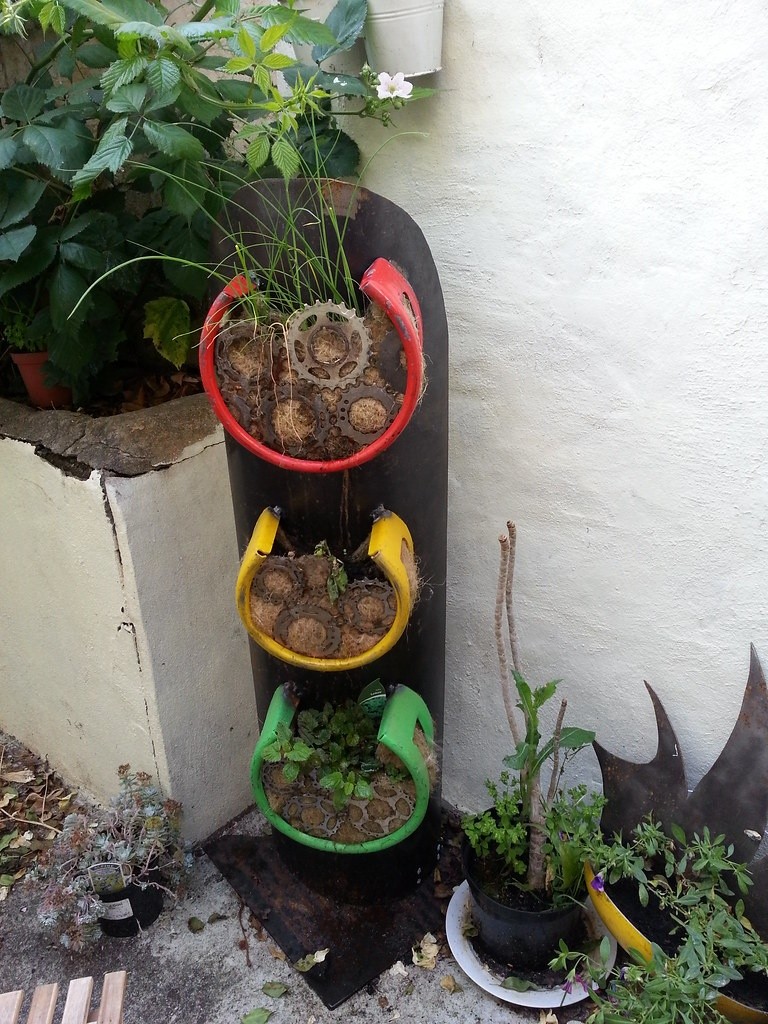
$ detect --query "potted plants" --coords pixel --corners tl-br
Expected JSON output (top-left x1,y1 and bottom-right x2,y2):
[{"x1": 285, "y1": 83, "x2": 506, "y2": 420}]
[
  {"x1": 73, "y1": 24, "x2": 429, "y2": 472},
  {"x1": 457, "y1": 516, "x2": 614, "y2": 973},
  {"x1": 24, "y1": 761, "x2": 194, "y2": 953},
  {"x1": 252, "y1": 679, "x2": 435, "y2": 855},
  {"x1": 362, "y1": 0, "x2": 446, "y2": 77},
  {"x1": 0, "y1": 279, "x2": 71, "y2": 408}
]
